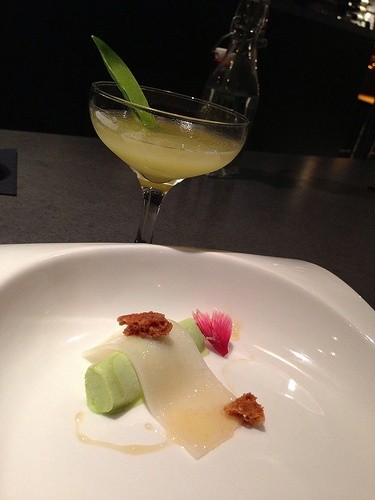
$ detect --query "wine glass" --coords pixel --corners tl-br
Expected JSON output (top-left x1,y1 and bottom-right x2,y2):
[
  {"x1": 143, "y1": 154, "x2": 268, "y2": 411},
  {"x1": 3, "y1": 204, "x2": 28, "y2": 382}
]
[{"x1": 88, "y1": 81, "x2": 249, "y2": 245}]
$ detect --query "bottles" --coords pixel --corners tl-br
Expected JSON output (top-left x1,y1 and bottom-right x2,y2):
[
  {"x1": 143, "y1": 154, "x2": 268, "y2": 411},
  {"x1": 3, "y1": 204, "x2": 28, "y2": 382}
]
[{"x1": 197, "y1": 2, "x2": 268, "y2": 179}]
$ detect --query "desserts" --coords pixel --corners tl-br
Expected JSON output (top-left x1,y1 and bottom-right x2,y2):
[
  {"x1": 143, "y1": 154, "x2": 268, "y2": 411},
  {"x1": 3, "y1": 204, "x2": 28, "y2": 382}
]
[{"x1": 83, "y1": 313, "x2": 266, "y2": 460}]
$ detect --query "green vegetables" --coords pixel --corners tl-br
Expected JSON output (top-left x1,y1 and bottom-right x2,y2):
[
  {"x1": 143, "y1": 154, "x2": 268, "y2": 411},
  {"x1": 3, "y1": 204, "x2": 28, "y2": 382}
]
[{"x1": 91, "y1": 34, "x2": 166, "y2": 135}]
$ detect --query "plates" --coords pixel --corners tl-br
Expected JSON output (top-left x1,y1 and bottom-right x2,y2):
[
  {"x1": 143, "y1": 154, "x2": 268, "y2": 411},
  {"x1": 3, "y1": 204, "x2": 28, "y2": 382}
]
[{"x1": 0, "y1": 241, "x2": 375, "y2": 500}]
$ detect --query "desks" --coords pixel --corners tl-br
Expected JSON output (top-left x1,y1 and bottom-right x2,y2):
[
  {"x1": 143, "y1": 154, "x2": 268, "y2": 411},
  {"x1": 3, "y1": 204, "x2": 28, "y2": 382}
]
[{"x1": 1, "y1": 129, "x2": 375, "y2": 311}]
